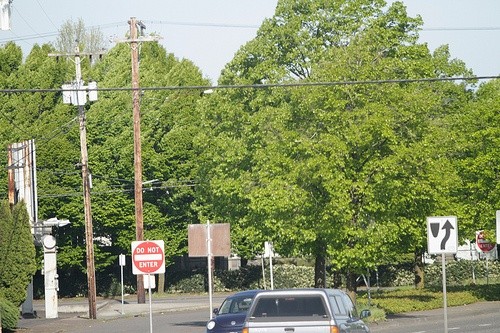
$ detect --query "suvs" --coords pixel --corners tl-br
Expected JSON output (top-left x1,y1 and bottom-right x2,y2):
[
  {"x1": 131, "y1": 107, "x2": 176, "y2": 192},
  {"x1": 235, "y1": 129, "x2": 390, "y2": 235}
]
[{"x1": 243, "y1": 288, "x2": 371, "y2": 333}]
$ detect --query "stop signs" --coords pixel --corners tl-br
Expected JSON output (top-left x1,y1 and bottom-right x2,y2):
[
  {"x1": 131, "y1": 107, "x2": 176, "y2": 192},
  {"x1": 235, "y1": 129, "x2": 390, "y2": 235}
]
[
  {"x1": 131, "y1": 239, "x2": 165, "y2": 275},
  {"x1": 476, "y1": 230, "x2": 496, "y2": 254}
]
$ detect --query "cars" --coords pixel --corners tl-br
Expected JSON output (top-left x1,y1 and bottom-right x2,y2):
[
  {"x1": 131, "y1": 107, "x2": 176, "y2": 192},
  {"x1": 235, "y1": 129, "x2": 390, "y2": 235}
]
[{"x1": 206, "y1": 291, "x2": 271, "y2": 333}]
